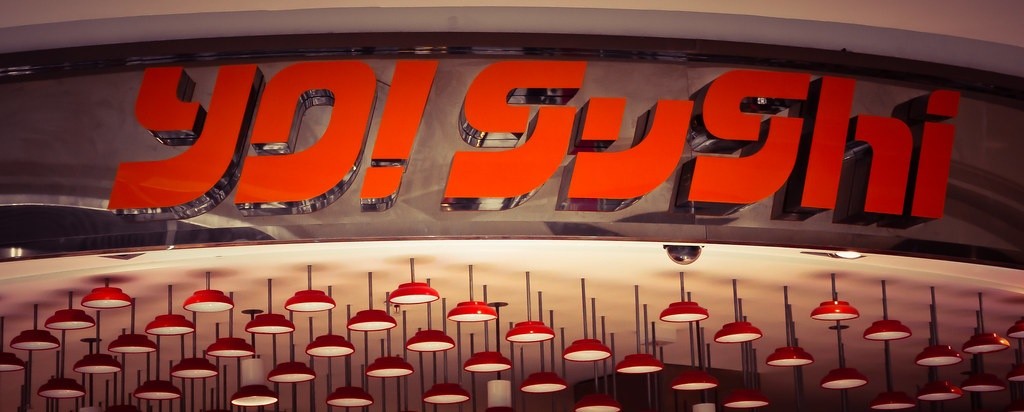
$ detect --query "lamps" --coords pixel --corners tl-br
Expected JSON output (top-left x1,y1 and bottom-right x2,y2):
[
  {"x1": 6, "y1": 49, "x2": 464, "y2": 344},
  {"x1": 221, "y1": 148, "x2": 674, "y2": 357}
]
[{"x1": 0, "y1": 259, "x2": 1024, "y2": 412}]
